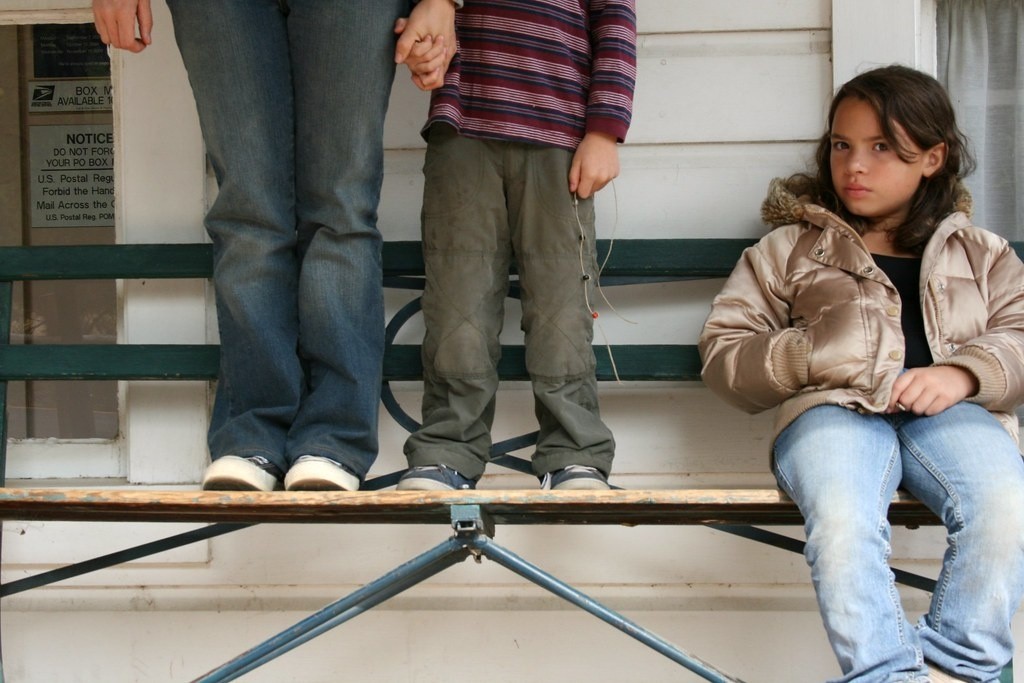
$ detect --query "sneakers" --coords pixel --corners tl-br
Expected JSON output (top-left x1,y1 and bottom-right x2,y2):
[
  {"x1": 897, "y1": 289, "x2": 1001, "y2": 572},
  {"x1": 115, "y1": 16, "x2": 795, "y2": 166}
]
[
  {"x1": 396, "y1": 465, "x2": 474, "y2": 491},
  {"x1": 540, "y1": 463, "x2": 609, "y2": 490},
  {"x1": 285, "y1": 452, "x2": 360, "y2": 493},
  {"x1": 203, "y1": 454, "x2": 280, "y2": 491}
]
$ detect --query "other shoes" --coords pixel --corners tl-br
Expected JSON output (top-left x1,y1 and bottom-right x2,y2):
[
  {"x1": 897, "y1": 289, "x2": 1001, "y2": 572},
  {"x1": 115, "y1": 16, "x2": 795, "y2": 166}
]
[{"x1": 924, "y1": 661, "x2": 998, "y2": 683}]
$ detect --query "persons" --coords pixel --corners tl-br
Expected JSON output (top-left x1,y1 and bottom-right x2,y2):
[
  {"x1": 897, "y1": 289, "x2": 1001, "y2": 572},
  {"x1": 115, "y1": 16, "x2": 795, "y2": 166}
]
[
  {"x1": 698, "y1": 65, "x2": 1024, "y2": 683},
  {"x1": 92, "y1": 0, "x2": 463, "y2": 491},
  {"x1": 394, "y1": 0, "x2": 638, "y2": 489}
]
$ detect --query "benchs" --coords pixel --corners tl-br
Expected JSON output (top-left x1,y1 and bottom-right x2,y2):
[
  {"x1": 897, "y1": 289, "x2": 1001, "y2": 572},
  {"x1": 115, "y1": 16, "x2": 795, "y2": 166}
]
[{"x1": 0, "y1": 240, "x2": 1024, "y2": 683}]
